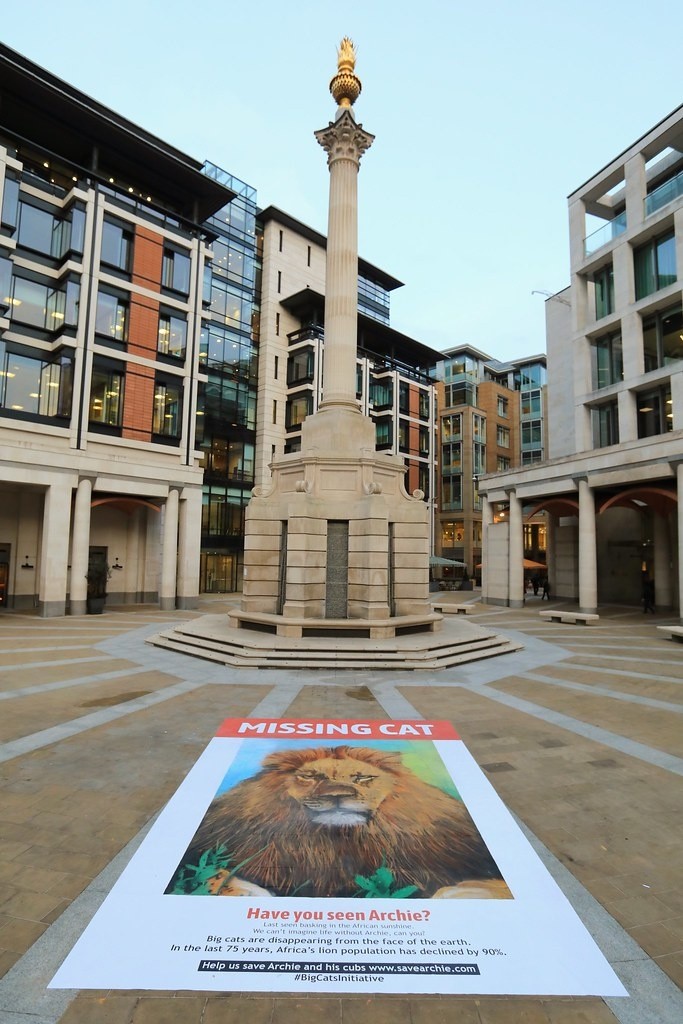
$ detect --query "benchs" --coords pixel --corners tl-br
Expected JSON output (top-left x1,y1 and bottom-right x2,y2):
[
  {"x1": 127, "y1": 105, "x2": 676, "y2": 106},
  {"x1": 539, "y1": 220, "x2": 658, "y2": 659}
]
[
  {"x1": 538, "y1": 610, "x2": 600, "y2": 626},
  {"x1": 431, "y1": 603, "x2": 476, "y2": 614},
  {"x1": 227, "y1": 608, "x2": 444, "y2": 639},
  {"x1": 656, "y1": 626, "x2": 683, "y2": 643}
]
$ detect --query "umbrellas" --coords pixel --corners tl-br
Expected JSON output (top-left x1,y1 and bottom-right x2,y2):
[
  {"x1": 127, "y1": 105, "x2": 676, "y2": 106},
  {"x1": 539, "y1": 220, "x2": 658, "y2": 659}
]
[
  {"x1": 428, "y1": 556, "x2": 467, "y2": 570},
  {"x1": 475, "y1": 556, "x2": 549, "y2": 572}
]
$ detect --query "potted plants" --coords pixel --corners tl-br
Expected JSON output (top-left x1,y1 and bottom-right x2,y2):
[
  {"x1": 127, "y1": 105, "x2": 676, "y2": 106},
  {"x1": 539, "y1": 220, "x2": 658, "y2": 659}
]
[{"x1": 85, "y1": 559, "x2": 112, "y2": 614}]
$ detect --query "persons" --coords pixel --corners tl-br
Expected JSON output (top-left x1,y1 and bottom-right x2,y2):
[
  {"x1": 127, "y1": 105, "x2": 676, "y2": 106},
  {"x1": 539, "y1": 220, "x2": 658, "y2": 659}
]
[
  {"x1": 639, "y1": 577, "x2": 656, "y2": 615},
  {"x1": 541, "y1": 577, "x2": 551, "y2": 601},
  {"x1": 531, "y1": 573, "x2": 539, "y2": 596}
]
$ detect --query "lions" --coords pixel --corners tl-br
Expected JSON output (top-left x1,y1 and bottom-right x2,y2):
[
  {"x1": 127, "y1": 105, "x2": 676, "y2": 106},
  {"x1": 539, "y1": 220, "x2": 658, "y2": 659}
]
[{"x1": 176, "y1": 746, "x2": 514, "y2": 898}]
